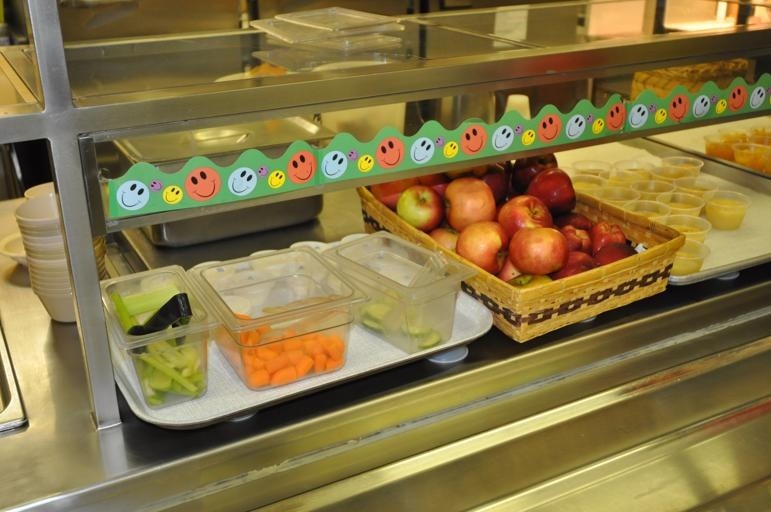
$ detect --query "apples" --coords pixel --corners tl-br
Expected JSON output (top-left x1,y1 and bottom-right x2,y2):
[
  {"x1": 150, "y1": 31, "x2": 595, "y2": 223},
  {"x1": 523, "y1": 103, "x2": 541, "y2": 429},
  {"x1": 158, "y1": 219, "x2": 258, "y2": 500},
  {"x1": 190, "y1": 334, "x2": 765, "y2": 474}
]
[{"x1": 370, "y1": 153, "x2": 639, "y2": 290}]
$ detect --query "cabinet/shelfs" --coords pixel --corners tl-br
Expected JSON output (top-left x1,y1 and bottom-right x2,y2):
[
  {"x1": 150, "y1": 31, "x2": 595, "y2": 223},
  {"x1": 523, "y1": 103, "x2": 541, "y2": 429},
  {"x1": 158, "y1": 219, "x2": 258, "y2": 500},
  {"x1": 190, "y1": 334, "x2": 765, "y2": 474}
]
[{"x1": 0, "y1": 0, "x2": 770, "y2": 511}]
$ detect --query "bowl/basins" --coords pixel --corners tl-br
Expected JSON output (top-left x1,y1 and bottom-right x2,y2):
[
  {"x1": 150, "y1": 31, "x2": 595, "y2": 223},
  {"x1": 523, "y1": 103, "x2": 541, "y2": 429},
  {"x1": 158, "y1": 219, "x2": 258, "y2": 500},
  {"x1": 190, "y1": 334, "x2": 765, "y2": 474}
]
[
  {"x1": 0, "y1": 234, "x2": 26, "y2": 268},
  {"x1": 569, "y1": 152, "x2": 750, "y2": 275},
  {"x1": 14, "y1": 182, "x2": 106, "y2": 325},
  {"x1": 704, "y1": 124, "x2": 771, "y2": 178}
]
